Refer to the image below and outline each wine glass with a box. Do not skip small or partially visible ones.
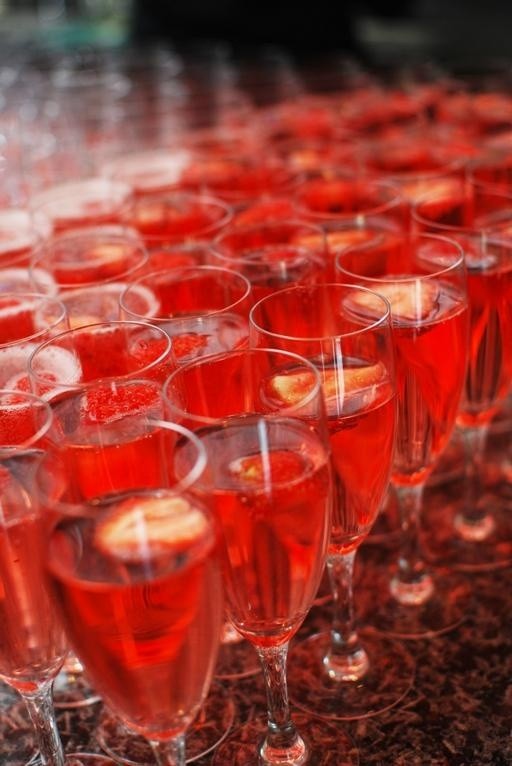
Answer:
[164,349,362,766]
[28,318,176,494]
[332,231,476,640]
[0,79,264,428]
[159,89,512,440]
[0,445,117,766]
[0,389,68,510]
[30,423,225,766]
[246,283,416,720]
